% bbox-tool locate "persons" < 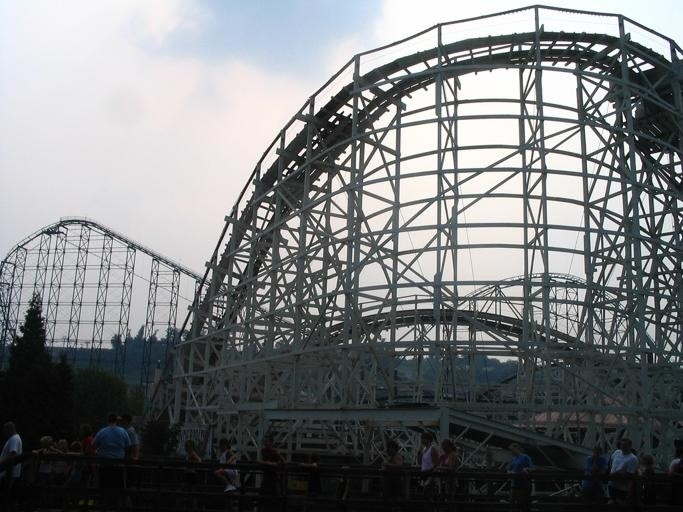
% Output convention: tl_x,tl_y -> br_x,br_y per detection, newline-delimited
1,413 -> 683,512
